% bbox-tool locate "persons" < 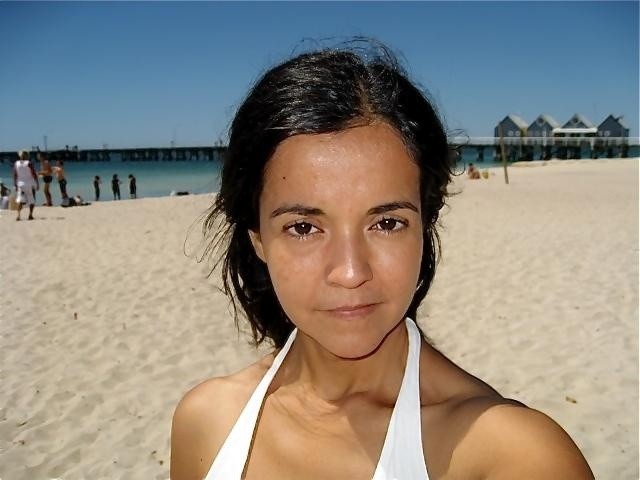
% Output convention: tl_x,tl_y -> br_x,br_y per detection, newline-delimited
128,173 -> 137,199
0,192 -> 10,209
52,158 -> 69,199
93,175 -> 101,201
166,33 -> 596,480
111,174 -> 123,200
466,162 -> 480,179
0,182 -> 12,197
11,147 -> 40,221
60,195 -> 90,208
36,150 -> 53,206
